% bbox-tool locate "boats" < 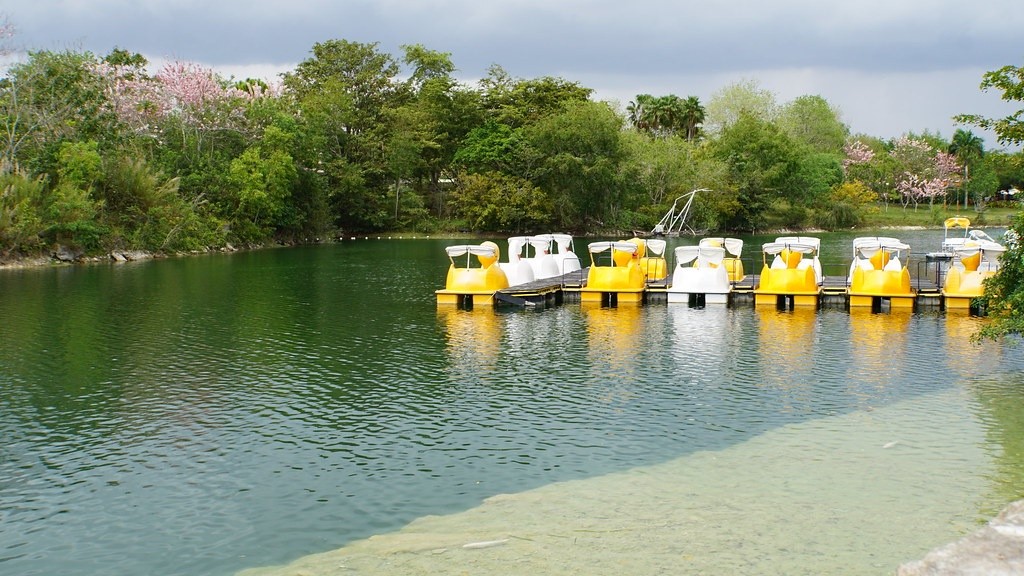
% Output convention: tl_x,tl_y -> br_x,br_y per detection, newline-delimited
667,237 -> 745,311
843,236 -> 919,316
942,251 -> 1002,318
433,233 -> 582,309
579,237 -> 668,305
753,235 -> 824,314
